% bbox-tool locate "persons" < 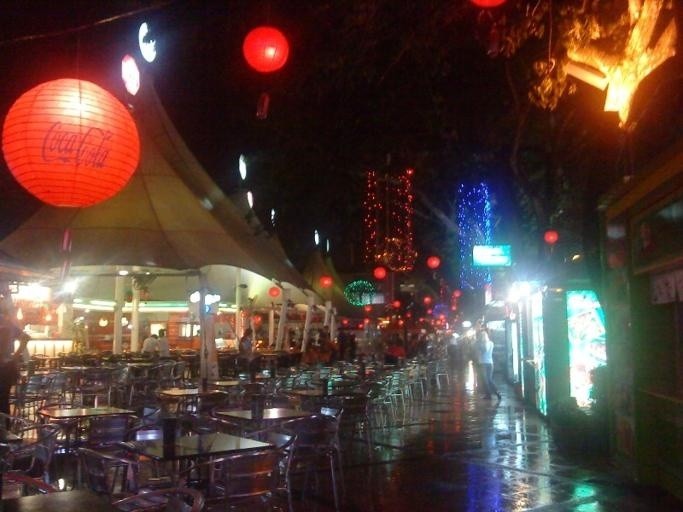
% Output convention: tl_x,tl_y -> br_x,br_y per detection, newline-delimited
375,333 -> 424,364
238,328 -> 254,371
0,295 -> 29,430
156,329 -> 168,359
141,335 -> 159,357
475,329 -> 501,400
290,326 -> 359,361
